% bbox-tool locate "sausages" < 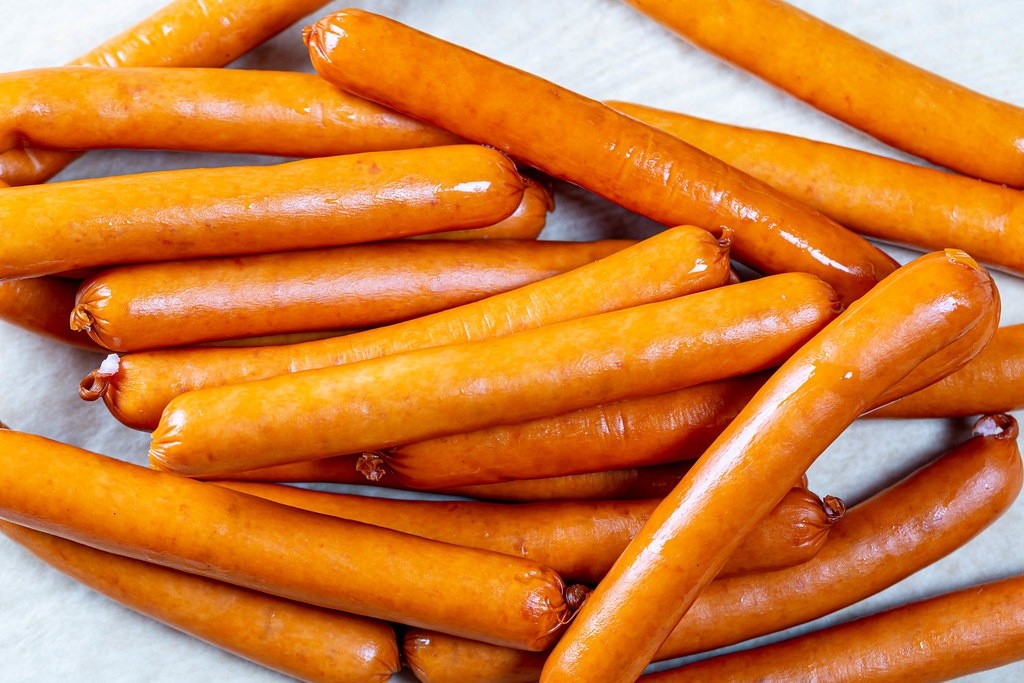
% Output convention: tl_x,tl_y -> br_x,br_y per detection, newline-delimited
0,0 -> 1024,683
